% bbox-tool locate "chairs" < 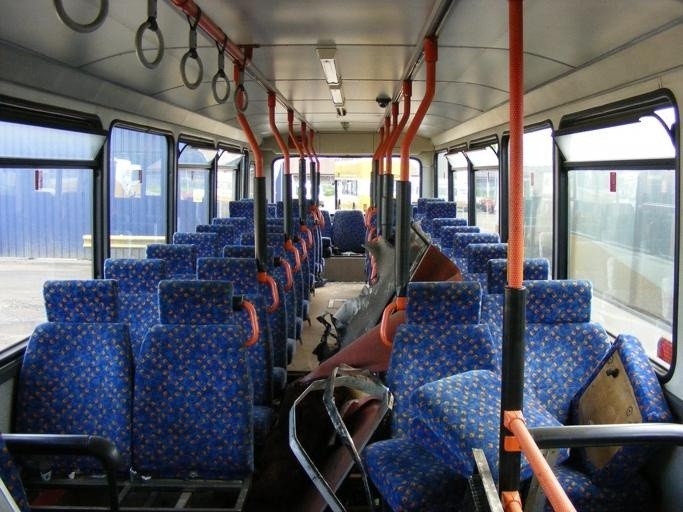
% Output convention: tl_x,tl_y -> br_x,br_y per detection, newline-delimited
333,209 -> 366,257
